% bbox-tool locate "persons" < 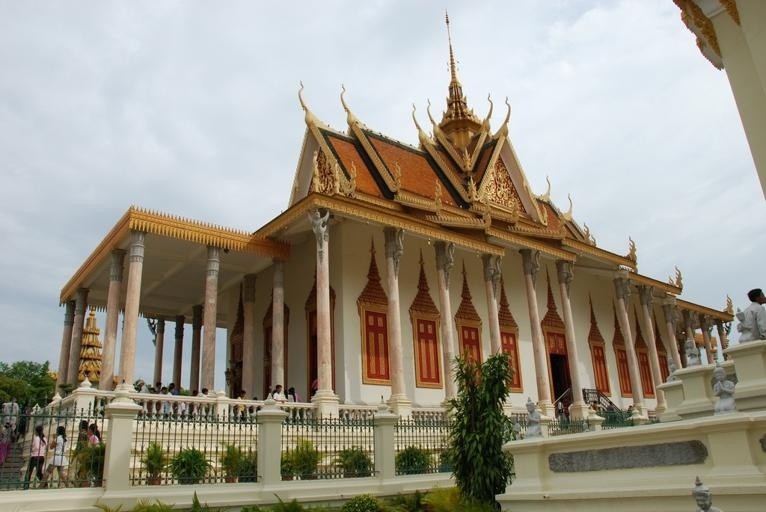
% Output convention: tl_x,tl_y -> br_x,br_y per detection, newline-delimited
685,338 -> 700,365
664,355 -> 677,380
713,367 -> 735,411
524,396 -> 541,432
738,288 -> 766,344
692,476 -> 723,512
